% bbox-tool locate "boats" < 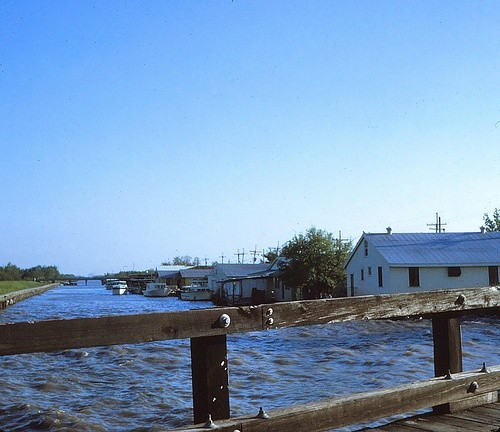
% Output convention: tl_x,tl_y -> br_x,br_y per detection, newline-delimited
175,285 -> 214,300
141,282 -> 177,296
62,280 -> 77,286
102,275 -> 157,295
